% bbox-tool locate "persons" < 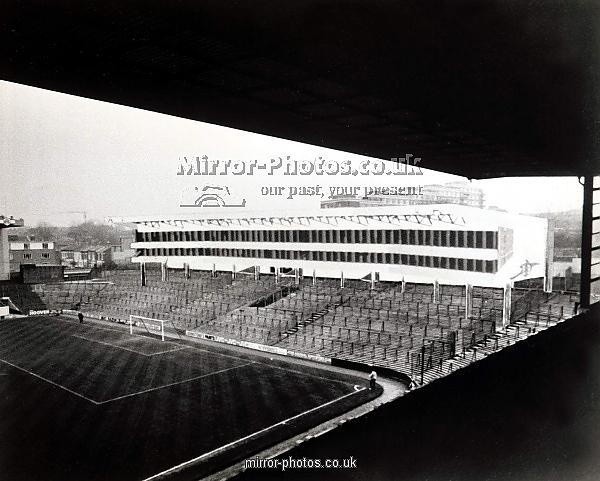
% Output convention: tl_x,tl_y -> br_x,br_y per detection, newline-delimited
368,369 -> 377,391
78,312 -> 83,323
48,310 -> 53,317
54,310 -> 60,316
